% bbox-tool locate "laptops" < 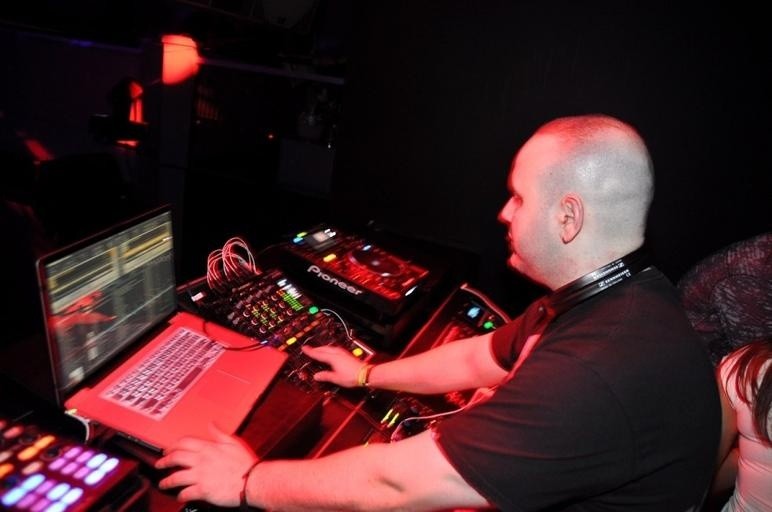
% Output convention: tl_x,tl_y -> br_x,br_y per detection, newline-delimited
35,202 -> 290,452
